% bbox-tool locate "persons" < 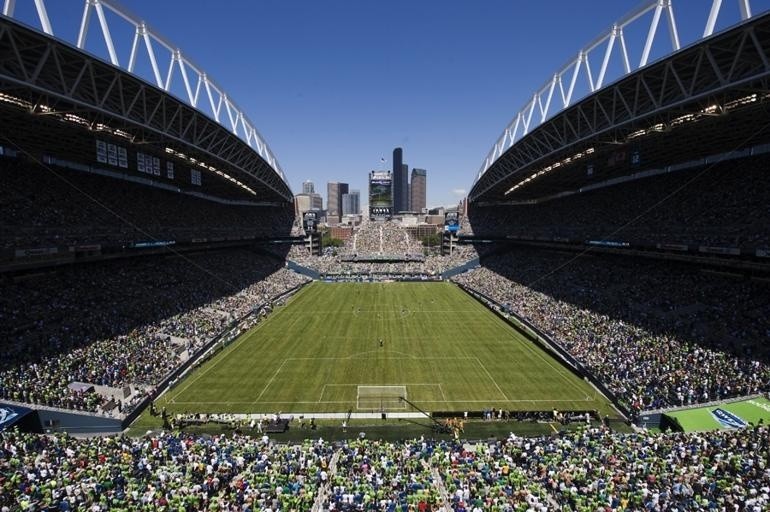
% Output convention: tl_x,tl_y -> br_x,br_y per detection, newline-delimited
0,160 -> 770,512
